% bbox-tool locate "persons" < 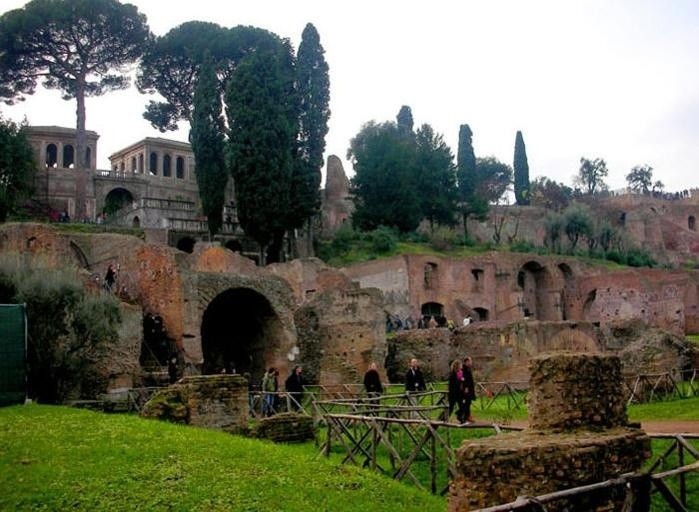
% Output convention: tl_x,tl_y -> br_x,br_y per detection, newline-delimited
168,357 -> 178,384
362,362 -> 383,416
455,356 -> 477,422
143,312 -> 170,366
405,358 -> 427,419
387,314 -> 473,331
439,358 -> 476,423
104,265 -> 116,289
49,208 -> 108,223
206,351 -> 304,417
